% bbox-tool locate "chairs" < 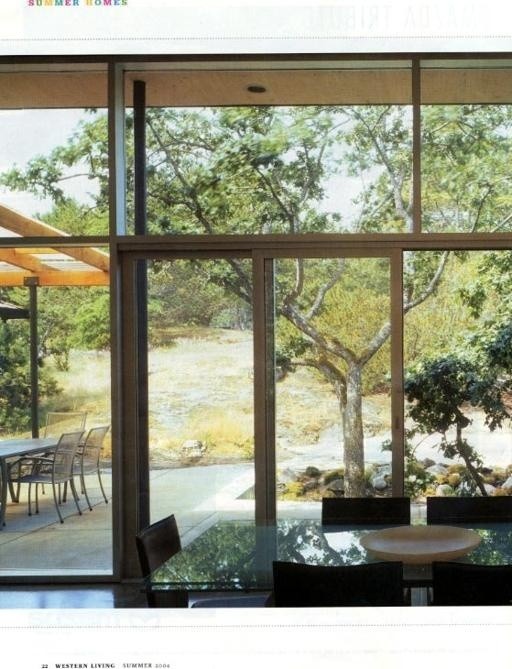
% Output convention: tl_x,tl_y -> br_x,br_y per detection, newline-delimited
0,411 -> 111,528
134,493 -> 510,605
